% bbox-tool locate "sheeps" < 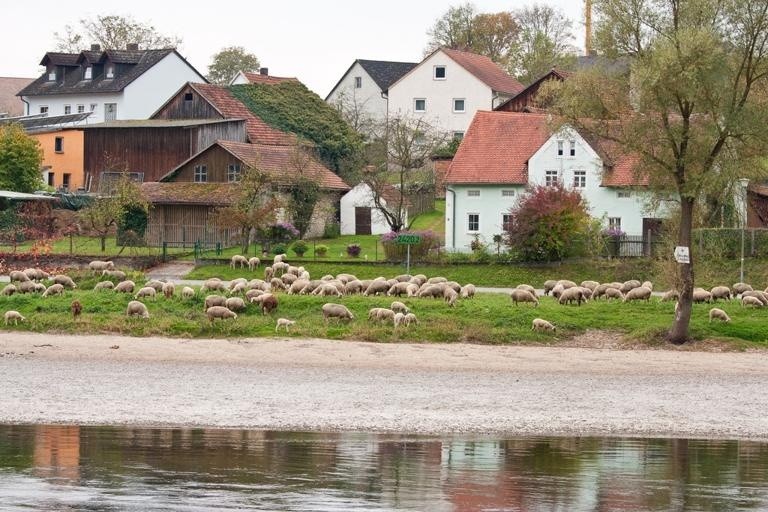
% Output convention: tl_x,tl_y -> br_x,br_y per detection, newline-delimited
230,255 -> 249,270
88,260 -> 114,270
510,280 -> 768,309
4,311 -> 26,327
274,254 -> 287,263
368,301 -> 418,329
71,299 -> 82,320
127,301 -> 149,321
200,277 -> 279,327
532,318 -> 556,333
322,303 -> 354,326
709,308 -> 731,322
265,262 -> 475,307
0,269 -> 174,302
247,257 -> 260,270
276,318 -> 296,333
182,287 -> 195,299
